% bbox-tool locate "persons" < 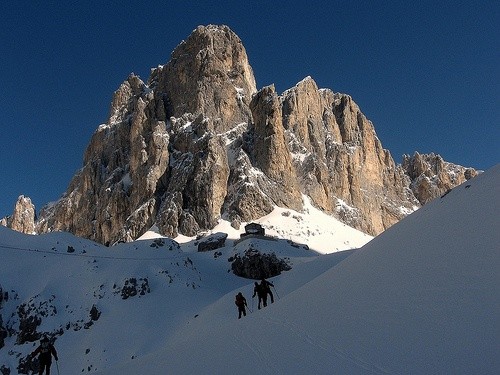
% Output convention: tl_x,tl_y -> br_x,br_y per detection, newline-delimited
252,281 -> 267,310
235,292 -> 247,319
259,277 -> 274,307
